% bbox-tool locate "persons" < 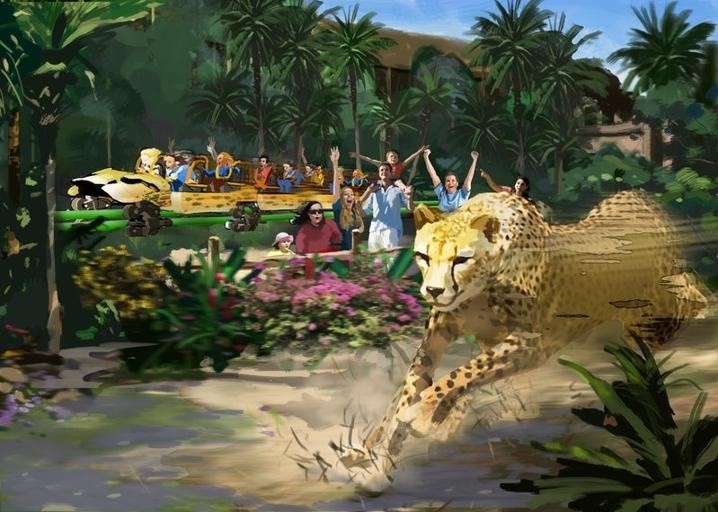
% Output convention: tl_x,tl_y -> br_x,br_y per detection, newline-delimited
421,145 -> 479,214
288,200 -> 343,254
139,134 -> 368,194
264,230 -> 295,257
348,143 -> 430,205
328,143 -> 365,251
358,160 -> 413,253
478,167 -> 530,203
